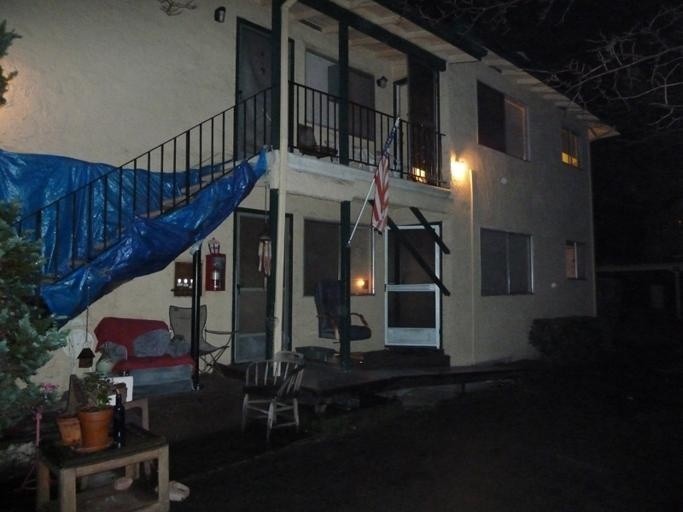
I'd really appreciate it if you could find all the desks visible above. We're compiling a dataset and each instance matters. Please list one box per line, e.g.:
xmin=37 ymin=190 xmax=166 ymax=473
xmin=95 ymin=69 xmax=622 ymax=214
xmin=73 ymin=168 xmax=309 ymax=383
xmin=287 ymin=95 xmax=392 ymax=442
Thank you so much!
xmin=35 ymin=423 xmax=170 ymax=512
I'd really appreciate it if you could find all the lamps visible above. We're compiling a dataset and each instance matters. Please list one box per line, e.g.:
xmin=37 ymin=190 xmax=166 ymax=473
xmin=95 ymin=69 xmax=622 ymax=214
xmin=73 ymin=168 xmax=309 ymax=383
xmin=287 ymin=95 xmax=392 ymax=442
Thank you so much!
xmin=257 ymin=233 xmax=272 ymax=278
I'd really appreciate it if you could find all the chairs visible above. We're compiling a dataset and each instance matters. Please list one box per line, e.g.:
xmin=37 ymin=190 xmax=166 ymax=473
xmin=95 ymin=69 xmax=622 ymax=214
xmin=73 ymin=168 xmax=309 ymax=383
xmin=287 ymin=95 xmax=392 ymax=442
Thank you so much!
xmin=297 ymin=123 xmax=338 ymax=159
xmin=240 ymin=351 xmax=309 ymax=442
xmin=169 ymin=305 xmax=235 ymax=379
xmin=314 ymin=279 xmax=371 ymax=367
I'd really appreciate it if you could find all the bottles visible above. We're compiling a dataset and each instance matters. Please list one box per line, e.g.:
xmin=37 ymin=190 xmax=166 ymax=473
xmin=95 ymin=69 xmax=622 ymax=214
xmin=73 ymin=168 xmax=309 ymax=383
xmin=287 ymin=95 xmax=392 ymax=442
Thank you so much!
xmin=114 ymin=394 xmax=125 ymax=449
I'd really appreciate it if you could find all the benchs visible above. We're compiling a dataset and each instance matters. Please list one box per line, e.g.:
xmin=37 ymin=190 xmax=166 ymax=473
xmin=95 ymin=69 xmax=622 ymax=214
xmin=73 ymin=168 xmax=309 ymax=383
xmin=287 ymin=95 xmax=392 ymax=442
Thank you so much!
xmin=94 ymin=317 xmax=193 ymax=397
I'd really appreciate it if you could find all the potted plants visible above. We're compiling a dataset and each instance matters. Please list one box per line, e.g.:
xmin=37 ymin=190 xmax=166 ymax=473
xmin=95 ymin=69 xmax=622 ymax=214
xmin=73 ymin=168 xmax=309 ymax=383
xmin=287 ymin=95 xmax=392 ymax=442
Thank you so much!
xmin=56 ymin=400 xmax=83 ymax=448
xmin=77 ymin=370 xmax=115 ymax=450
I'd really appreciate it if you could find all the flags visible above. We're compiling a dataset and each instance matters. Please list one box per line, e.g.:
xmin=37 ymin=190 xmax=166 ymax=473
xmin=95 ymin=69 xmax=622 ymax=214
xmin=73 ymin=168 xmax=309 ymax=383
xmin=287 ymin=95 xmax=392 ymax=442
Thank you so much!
xmin=371 ymin=118 xmax=400 ymax=235
